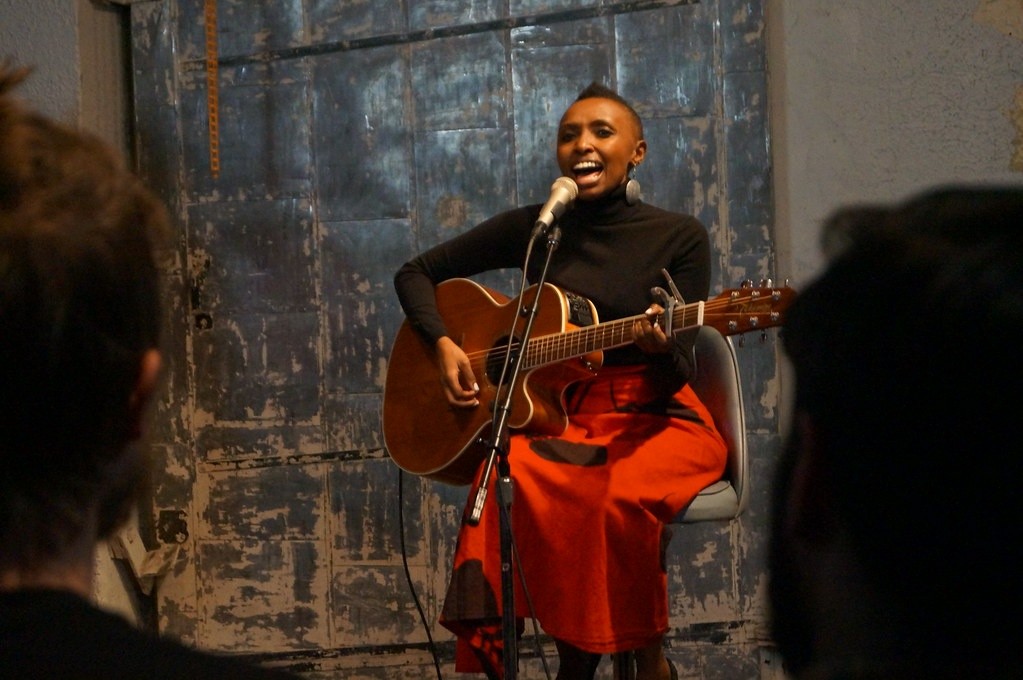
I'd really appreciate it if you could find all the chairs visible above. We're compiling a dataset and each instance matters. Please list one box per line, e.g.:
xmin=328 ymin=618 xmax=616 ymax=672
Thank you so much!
xmin=613 ymin=326 xmax=750 ymax=680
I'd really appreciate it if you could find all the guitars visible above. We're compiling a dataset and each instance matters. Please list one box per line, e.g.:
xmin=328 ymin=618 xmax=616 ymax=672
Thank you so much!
xmin=380 ymin=276 xmax=802 ymax=489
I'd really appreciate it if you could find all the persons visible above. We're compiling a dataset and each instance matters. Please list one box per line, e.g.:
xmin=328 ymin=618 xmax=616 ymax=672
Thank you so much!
xmin=394 ymin=82 xmax=729 ymax=680
xmin=765 ymin=184 xmax=1022 ymax=680
xmin=0 ymin=63 xmax=315 ymax=680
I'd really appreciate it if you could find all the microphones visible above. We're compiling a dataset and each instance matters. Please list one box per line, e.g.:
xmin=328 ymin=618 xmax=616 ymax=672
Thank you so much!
xmin=530 ymin=176 xmax=578 ymax=243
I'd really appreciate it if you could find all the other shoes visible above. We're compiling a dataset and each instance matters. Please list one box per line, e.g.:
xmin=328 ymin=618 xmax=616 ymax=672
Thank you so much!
xmin=556 ymin=653 xmax=601 ymax=680
xmin=666 ymin=657 xmax=678 ymax=680
